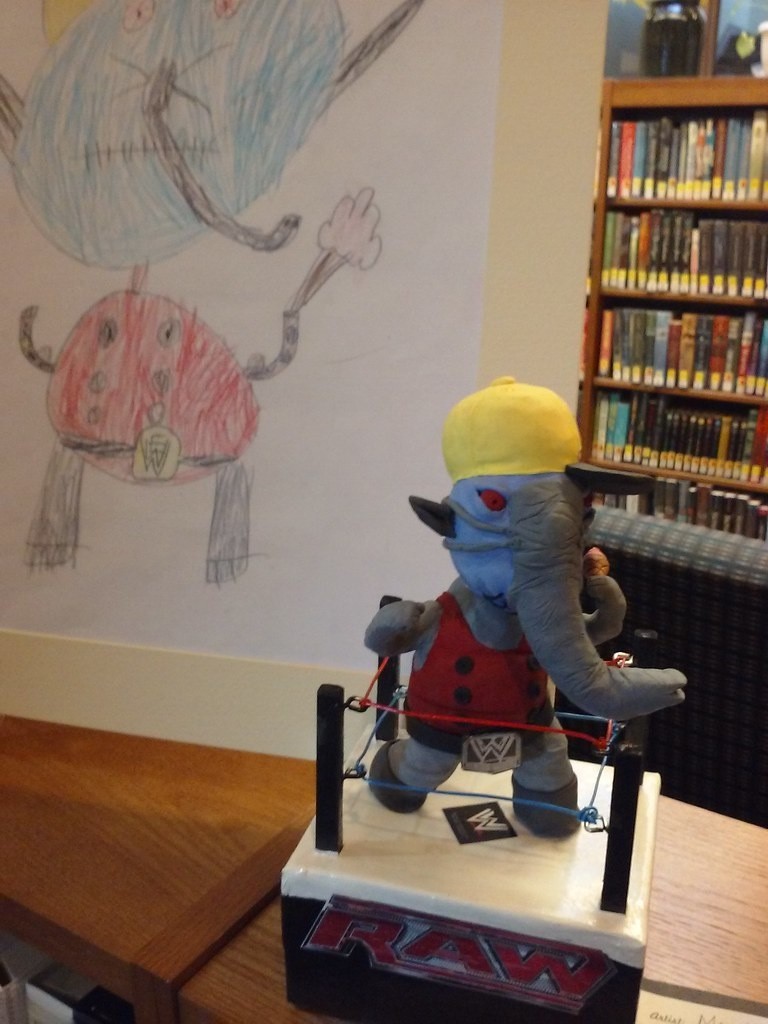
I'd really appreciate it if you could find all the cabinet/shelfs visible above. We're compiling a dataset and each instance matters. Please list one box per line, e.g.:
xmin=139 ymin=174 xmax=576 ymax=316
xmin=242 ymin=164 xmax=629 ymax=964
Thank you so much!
xmin=552 ymin=66 xmax=768 ymax=543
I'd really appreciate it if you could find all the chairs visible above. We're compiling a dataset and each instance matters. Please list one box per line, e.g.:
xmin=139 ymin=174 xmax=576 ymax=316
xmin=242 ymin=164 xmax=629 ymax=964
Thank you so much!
xmin=555 ymin=505 xmax=768 ymax=823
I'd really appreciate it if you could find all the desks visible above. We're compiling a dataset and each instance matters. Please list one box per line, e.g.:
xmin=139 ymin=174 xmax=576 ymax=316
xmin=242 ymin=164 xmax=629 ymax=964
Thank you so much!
xmin=0 ymin=708 xmax=768 ymax=1024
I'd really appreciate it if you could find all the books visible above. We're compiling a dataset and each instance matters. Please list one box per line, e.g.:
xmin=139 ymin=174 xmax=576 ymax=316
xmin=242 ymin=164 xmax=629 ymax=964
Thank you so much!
xmin=605 ymin=110 xmax=768 ymax=200
xmin=589 ymin=389 xmax=768 ymax=484
xmin=598 ymin=309 xmax=768 ymax=399
xmin=592 ymin=475 xmax=768 ymax=540
xmin=600 ymin=206 xmax=768 ymax=298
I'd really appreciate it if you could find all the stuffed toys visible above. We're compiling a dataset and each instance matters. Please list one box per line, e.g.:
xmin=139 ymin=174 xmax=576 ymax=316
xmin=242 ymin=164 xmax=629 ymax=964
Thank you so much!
xmin=364 ymin=372 xmax=689 ymax=835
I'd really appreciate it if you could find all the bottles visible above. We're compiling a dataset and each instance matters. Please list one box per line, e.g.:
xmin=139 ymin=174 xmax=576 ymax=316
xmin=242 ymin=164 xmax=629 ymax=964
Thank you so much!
xmin=638 ymin=0 xmax=707 ymax=78
